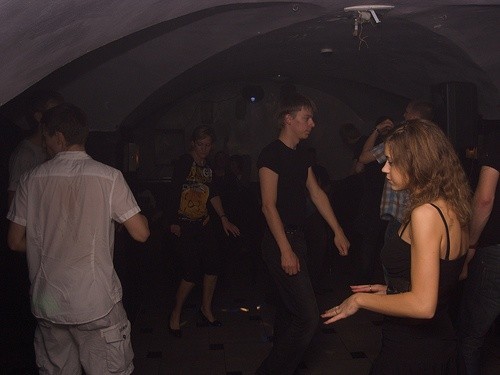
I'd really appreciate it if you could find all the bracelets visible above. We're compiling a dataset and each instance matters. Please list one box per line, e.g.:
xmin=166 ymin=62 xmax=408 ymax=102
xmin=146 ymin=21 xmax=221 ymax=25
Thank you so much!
xmin=375 ymin=128 xmax=381 ymax=133
xmin=221 ymin=215 xmax=224 ymax=217
xmin=470 ymin=245 xmax=477 ymax=249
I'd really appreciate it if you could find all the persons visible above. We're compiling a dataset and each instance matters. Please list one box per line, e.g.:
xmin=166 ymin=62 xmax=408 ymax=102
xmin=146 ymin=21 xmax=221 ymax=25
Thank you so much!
xmin=170 ymin=126 xmax=240 ymax=337
xmin=321 ymin=119 xmax=472 ymax=375
xmin=440 ymin=131 xmax=500 ymax=375
xmin=255 ymin=95 xmax=373 ymax=375
xmin=2 ymin=105 xmax=150 ymax=375
xmin=359 ymin=100 xmax=430 ymax=286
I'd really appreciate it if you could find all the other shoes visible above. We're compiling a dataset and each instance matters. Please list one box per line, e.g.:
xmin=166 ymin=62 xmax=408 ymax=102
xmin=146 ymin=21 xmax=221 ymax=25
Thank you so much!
xmin=198 ymin=307 xmax=221 ymax=328
xmin=168 ymin=319 xmax=182 ymax=338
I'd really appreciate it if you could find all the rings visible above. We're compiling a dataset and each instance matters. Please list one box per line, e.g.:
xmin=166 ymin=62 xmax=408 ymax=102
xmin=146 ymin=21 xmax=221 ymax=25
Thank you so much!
xmin=370 ymin=285 xmax=371 ymax=292
xmin=335 ymin=310 xmax=337 ymax=315
